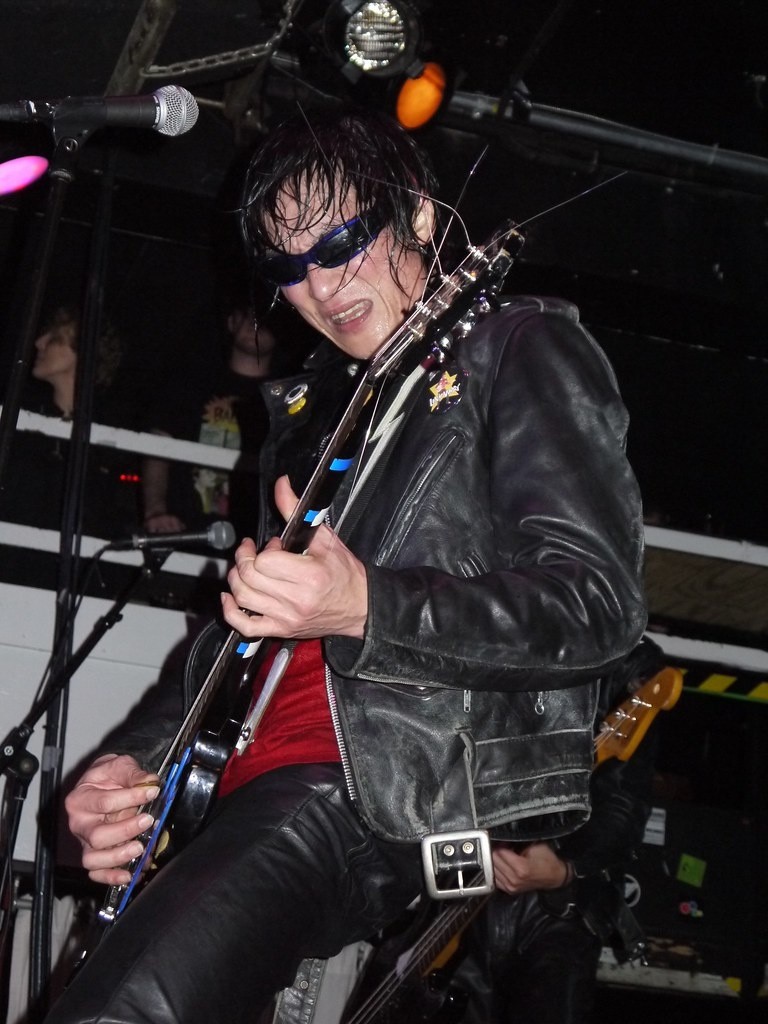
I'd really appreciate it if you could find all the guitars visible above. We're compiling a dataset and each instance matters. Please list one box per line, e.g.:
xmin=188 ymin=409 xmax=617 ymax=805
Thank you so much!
xmin=84 ymin=217 xmax=527 ymax=923
xmin=339 ymin=664 xmax=688 ymax=1023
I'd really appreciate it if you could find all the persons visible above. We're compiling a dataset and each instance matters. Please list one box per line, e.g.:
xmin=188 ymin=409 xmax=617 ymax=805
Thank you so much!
xmin=9 ymin=306 xmax=137 ymax=546
xmin=142 ymin=289 xmax=288 ymax=534
xmin=65 ymin=117 xmax=677 ymax=1024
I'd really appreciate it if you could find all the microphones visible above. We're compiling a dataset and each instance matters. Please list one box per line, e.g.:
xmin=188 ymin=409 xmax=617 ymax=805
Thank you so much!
xmin=0 ymin=84 xmax=199 ymax=136
xmin=111 ymin=520 xmax=236 ymax=551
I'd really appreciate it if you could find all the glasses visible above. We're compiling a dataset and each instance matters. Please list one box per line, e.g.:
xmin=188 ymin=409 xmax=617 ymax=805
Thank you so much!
xmin=262 ymin=191 xmax=415 ymax=287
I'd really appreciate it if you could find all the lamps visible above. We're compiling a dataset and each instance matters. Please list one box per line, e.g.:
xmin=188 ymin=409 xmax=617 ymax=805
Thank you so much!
xmin=310 ymin=0 xmax=456 ymax=139
